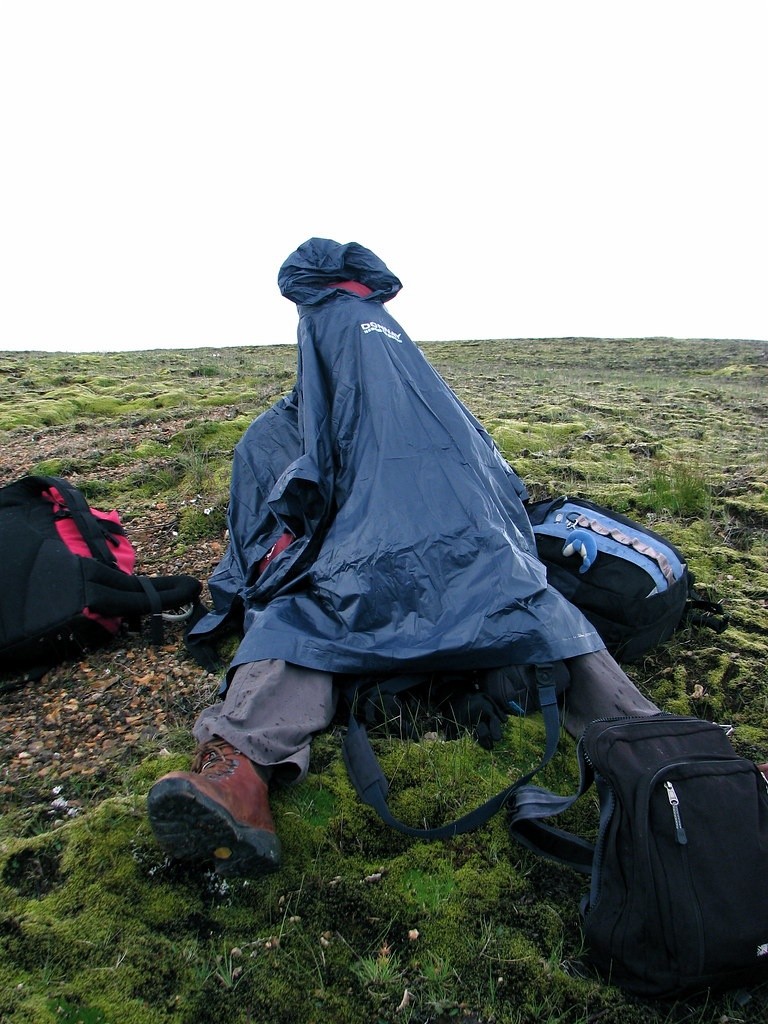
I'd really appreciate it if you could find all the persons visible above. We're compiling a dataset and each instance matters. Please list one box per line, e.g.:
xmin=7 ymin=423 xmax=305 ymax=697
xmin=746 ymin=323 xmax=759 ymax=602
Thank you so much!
xmin=147 ymin=280 xmax=768 ymax=876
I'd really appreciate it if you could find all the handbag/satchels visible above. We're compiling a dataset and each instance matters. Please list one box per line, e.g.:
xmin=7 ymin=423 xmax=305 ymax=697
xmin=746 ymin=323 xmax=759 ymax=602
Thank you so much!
xmin=342 ymin=659 xmax=570 ymax=841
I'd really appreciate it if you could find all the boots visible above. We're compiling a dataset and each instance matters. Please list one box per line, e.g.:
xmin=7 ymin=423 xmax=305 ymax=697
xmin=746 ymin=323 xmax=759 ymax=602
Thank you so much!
xmin=147 ymin=740 xmax=281 ymax=874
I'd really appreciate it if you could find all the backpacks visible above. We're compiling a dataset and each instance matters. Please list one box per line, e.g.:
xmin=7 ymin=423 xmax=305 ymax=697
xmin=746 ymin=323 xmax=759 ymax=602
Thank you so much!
xmin=529 ymin=494 xmax=692 ymax=667
xmin=505 ymin=712 xmax=768 ymax=1005
xmin=0 ymin=475 xmax=201 ymax=664
